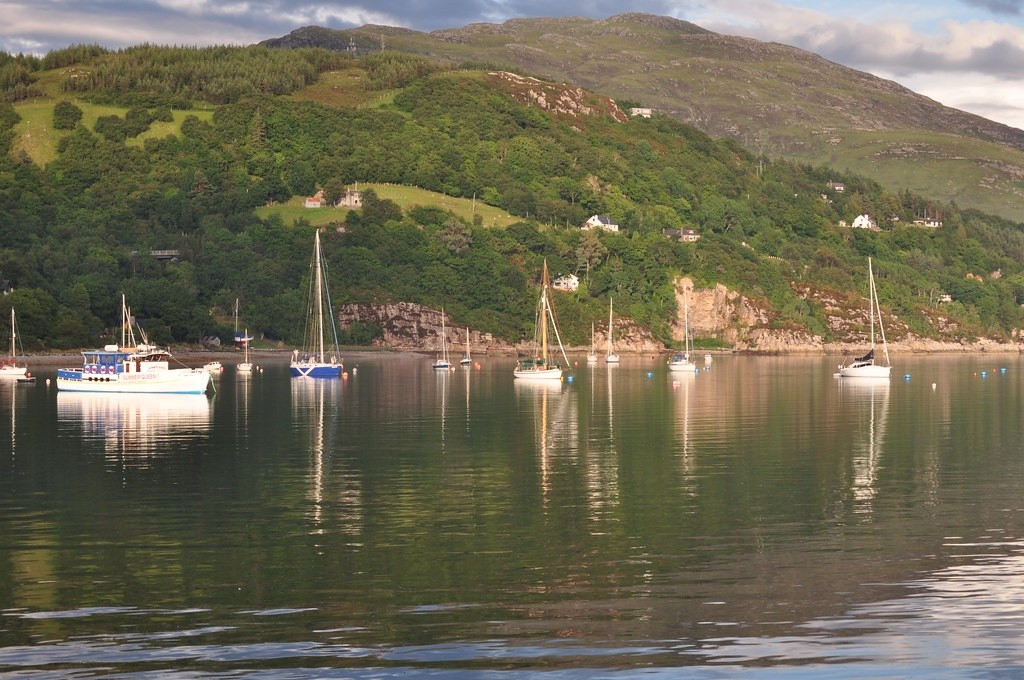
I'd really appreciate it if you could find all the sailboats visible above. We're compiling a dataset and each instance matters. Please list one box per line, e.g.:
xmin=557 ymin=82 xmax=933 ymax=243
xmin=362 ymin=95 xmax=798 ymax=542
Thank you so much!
xmin=516 ymin=255 xmax=562 ymax=370
xmin=585 ymin=321 xmax=597 ymax=361
xmin=0 ymin=305 xmax=37 ymax=383
xmin=56 ymin=295 xmax=210 ymax=393
xmin=236 ymin=328 xmax=253 ymax=371
xmin=432 ymin=308 xmax=452 ymax=371
xmin=289 ymin=228 xmax=344 ymax=378
xmin=459 ymin=326 xmax=472 ymax=365
xmin=667 ymin=280 xmax=696 ymax=371
xmin=604 ymin=296 xmax=619 ymax=362
xmin=511 ymin=287 xmax=563 ymax=378
xmin=135 ymin=321 xmax=172 ymax=360
xmin=231 ymin=299 xmax=254 ymax=342
xmin=838 ymin=255 xmax=891 ymax=378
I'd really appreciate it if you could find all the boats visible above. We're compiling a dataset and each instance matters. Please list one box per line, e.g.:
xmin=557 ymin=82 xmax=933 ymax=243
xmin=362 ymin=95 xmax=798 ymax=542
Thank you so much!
xmin=202 ymin=360 xmax=222 ymax=371
